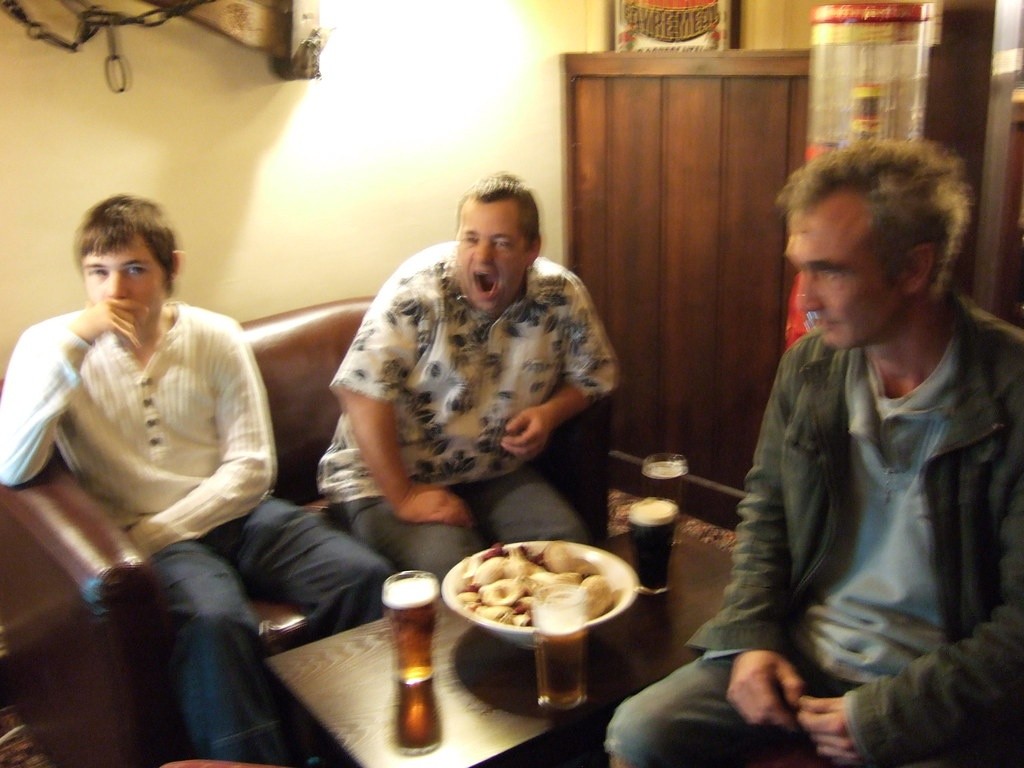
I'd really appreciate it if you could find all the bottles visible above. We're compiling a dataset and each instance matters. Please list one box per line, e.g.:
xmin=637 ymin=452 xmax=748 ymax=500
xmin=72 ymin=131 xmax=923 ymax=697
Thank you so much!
xmin=847 ymin=44 xmax=883 ymax=148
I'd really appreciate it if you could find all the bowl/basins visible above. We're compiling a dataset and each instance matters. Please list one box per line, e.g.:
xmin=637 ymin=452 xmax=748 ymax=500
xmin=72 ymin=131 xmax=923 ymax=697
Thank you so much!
xmin=441 ymin=540 xmax=640 ymax=649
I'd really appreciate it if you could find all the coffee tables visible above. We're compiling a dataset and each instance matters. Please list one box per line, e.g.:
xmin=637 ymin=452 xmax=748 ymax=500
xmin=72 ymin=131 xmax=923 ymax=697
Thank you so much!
xmin=265 ymin=493 xmax=778 ymax=767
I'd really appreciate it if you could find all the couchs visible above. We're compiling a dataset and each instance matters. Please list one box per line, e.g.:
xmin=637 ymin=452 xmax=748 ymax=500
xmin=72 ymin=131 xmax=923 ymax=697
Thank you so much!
xmin=0 ymin=295 xmax=608 ymax=767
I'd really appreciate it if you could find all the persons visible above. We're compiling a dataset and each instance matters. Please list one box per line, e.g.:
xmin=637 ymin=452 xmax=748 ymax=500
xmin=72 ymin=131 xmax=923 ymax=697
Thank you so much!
xmin=604 ymin=134 xmax=1024 ymax=768
xmin=0 ymin=196 xmax=392 ymax=768
xmin=316 ymin=171 xmax=618 ymax=586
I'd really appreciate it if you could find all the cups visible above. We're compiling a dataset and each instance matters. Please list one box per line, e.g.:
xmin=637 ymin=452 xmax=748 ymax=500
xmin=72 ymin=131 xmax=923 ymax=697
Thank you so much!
xmin=529 ymin=584 xmax=589 ymax=711
xmin=628 ymin=496 xmax=679 ymax=595
xmin=380 ymin=570 xmax=440 ymax=685
xmin=389 ymin=682 xmax=442 ymax=757
xmin=640 ymin=452 xmax=689 ymax=504
